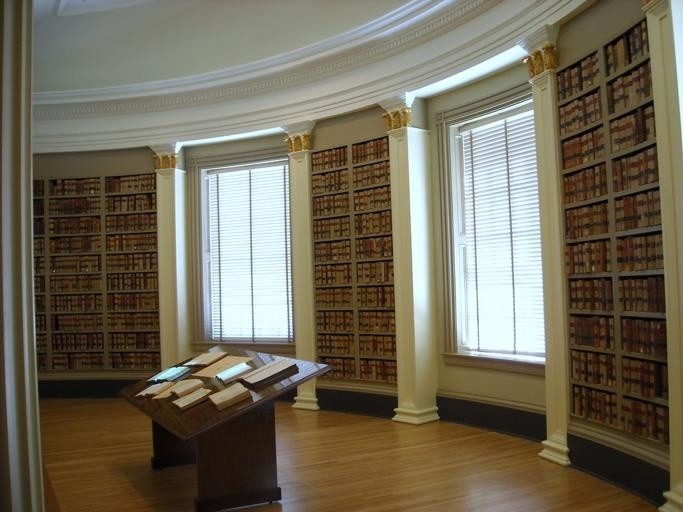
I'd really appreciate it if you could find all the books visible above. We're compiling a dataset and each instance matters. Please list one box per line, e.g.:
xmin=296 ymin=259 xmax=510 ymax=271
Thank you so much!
xmin=33 ymin=171 xmax=159 ymax=373
xmin=554 ymin=19 xmax=670 ymax=449
xmin=135 ymin=353 xmax=298 ymax=413
xmin=312 ymin=137 xmax=396 ymax=384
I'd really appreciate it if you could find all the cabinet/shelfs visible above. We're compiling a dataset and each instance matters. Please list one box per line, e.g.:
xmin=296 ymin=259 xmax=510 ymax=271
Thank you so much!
xmin=308 ymin=134 xmax=397 ymax=387
xmin=31 ymin=169 xmax=161 ymax=371
xmin=553 ymin=16 xmax=668 ymax=458
